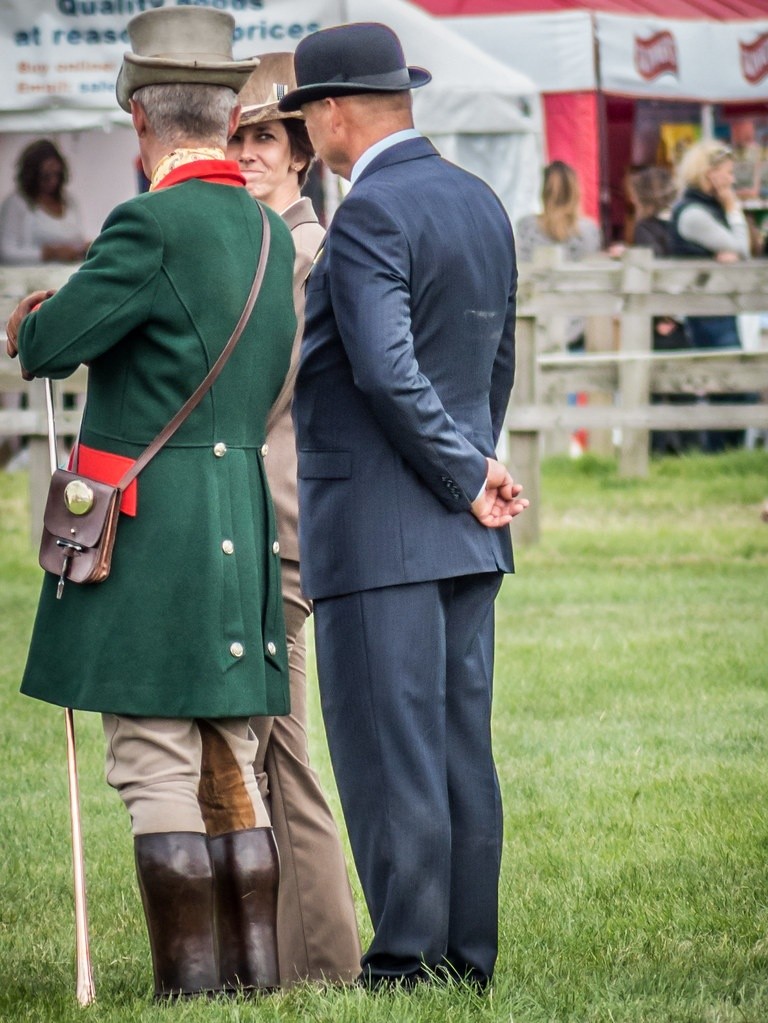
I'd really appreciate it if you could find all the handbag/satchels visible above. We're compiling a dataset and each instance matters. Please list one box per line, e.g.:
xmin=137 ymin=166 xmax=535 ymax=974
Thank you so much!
xmin=38 ymin=468 xmax=123 ymax=600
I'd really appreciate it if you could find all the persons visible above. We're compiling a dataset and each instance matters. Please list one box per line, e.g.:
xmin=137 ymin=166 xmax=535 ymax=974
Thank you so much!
xmin=354 ymin=1007 xmax=358 ymax=1010
xmin=0 ymin=7 xmax=768 ymax=1010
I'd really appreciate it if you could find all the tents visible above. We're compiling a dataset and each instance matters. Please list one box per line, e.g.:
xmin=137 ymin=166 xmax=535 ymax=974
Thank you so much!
xmin=323 ymin=0 xmax=768 ymax=254
xmin=0 ymin=0 xmax=546 ymax=216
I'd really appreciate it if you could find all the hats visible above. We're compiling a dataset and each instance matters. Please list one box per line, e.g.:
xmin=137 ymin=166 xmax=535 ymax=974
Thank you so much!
xmin=237 ymin=51 xmax=306 ymax=126
xmin=277 ymin=22 xmax=432 ymax=113
xmin=114 ymin=6 xmax=262 ymax=115
